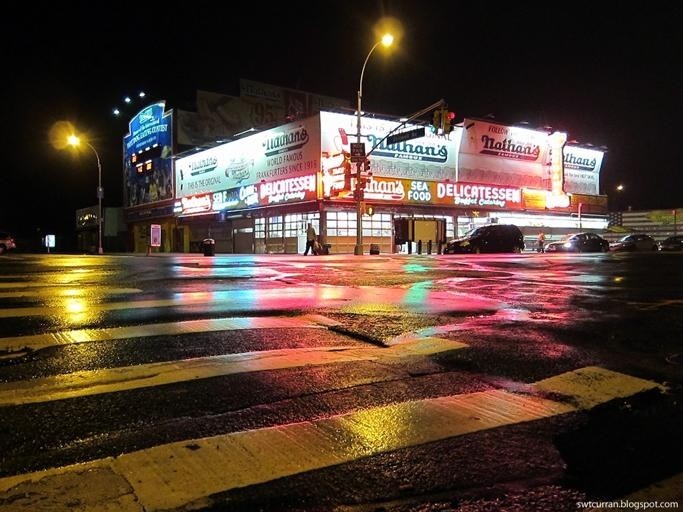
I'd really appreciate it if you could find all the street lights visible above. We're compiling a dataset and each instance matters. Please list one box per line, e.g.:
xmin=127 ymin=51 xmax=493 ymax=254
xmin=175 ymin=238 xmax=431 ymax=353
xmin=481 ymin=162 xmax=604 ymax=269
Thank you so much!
xmin=354 ymin=33 xmax=394 ymax=256
xmin=68 ymin=136 xmax=103 ymax=255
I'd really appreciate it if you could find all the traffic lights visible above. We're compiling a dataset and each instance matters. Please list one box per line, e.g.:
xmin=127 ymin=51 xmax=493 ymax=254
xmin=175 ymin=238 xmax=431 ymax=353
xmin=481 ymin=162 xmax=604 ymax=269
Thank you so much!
xmin=441 ymin=109 xmax=455 ymax=135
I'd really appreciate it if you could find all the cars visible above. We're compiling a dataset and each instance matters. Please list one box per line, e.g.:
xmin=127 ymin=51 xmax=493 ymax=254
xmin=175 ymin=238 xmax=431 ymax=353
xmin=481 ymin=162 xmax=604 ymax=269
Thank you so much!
xmin=544 ymin=232 xmax=683 ymax=253
xmin=0 ymin=231 xmax=16 ymax=254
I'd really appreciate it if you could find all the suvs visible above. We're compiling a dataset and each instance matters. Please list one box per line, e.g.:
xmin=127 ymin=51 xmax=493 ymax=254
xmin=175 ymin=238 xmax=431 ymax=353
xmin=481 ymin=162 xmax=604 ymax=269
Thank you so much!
xmin=444 ymin=224 xmax=526 ymax=254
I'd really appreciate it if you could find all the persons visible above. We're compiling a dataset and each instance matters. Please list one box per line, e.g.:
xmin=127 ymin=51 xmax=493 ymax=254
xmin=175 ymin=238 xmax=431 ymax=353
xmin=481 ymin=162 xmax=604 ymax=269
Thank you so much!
xmin=536 ymin=230 xmax=545 ymax=253
xmin=303 ymin=223 xmax=318 ymax=256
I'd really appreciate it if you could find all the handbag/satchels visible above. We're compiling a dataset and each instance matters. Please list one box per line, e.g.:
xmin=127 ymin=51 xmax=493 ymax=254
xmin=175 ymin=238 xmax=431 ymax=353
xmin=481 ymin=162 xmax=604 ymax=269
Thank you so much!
xmin=313 ymin=242 xmax=319 ymax=252
